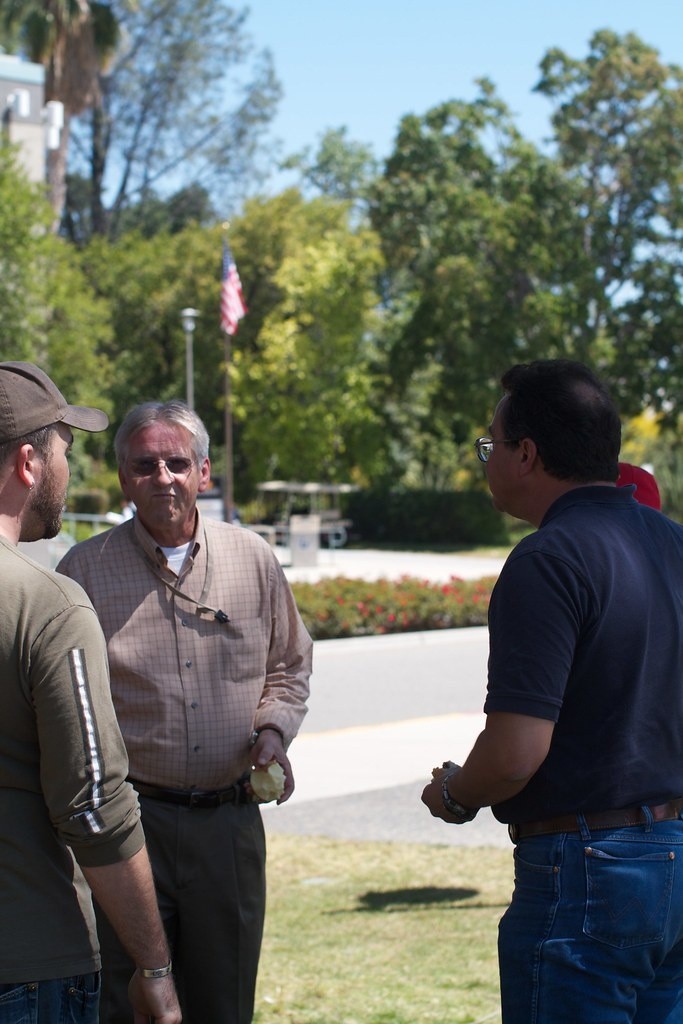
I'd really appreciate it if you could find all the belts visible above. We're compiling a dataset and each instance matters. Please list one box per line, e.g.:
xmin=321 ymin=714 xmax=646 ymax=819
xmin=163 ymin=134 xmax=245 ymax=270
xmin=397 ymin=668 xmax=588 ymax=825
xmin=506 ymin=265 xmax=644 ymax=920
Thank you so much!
xmin=508 ymin=799 xmax=683 ymax=841
xmin=127 ymin=776 xmax=249 ymax=808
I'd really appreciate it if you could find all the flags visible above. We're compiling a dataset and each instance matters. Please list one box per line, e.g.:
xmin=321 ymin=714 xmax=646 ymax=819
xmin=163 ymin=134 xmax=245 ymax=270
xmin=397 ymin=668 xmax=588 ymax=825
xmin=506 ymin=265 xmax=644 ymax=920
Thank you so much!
xmin=220 ymin=243 xmax=248 ymax=338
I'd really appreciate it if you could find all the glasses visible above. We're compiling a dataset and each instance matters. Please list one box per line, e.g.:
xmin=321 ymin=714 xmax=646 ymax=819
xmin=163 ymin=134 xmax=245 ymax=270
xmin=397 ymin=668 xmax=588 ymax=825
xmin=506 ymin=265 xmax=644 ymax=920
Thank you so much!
xmin=476 ymin=436 xmax=519 ymax=461
xmin=123 ymin=453 xmax=203 ymax=476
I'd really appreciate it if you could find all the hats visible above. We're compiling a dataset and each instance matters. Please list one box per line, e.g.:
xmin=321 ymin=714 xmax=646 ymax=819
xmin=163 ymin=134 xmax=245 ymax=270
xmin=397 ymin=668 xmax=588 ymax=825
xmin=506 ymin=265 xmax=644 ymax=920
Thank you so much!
xmin=617 ymin=463 xmax=661 ymax=511
xmin=0 ymin=360 xmax=110 ymax=442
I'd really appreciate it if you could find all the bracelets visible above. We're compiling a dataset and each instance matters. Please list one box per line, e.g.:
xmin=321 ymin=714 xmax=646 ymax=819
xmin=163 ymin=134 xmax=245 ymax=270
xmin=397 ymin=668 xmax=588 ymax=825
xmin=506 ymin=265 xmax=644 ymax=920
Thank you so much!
xmin=141 ymin=957 xmax=172 ymax=977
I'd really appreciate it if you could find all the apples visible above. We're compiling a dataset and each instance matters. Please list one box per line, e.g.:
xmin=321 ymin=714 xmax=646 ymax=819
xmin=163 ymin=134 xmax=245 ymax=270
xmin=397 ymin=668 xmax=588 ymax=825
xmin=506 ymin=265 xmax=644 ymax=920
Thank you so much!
xmin=249 ymin=761 xmax=286 ymax=800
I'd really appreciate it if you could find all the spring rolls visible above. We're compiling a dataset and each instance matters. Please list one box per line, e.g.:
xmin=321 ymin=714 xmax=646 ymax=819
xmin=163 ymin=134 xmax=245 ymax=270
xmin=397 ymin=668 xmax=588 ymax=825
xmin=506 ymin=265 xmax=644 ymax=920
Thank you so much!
xmin=432 ymin=761 xmax=460 ymax=781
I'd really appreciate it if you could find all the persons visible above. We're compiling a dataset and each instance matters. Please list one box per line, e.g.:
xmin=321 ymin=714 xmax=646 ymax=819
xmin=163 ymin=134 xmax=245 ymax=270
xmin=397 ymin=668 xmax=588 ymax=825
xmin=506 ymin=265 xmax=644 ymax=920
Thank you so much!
xmin=56 ymin=402 xmax=312 ymax=1024
xmin=420 ymin=356 xmax=683 ymax=1024
xmin=0 ymin=359 xmax=183 ymax=1024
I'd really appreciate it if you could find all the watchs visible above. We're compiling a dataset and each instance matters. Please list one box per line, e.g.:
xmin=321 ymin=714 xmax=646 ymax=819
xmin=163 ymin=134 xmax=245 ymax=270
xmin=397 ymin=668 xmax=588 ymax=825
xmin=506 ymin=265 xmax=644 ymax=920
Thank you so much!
xmin=439 ymin=774 xmax=480 ymax=822
xmin=248 ymin=726 xmax=285 ymax=746
